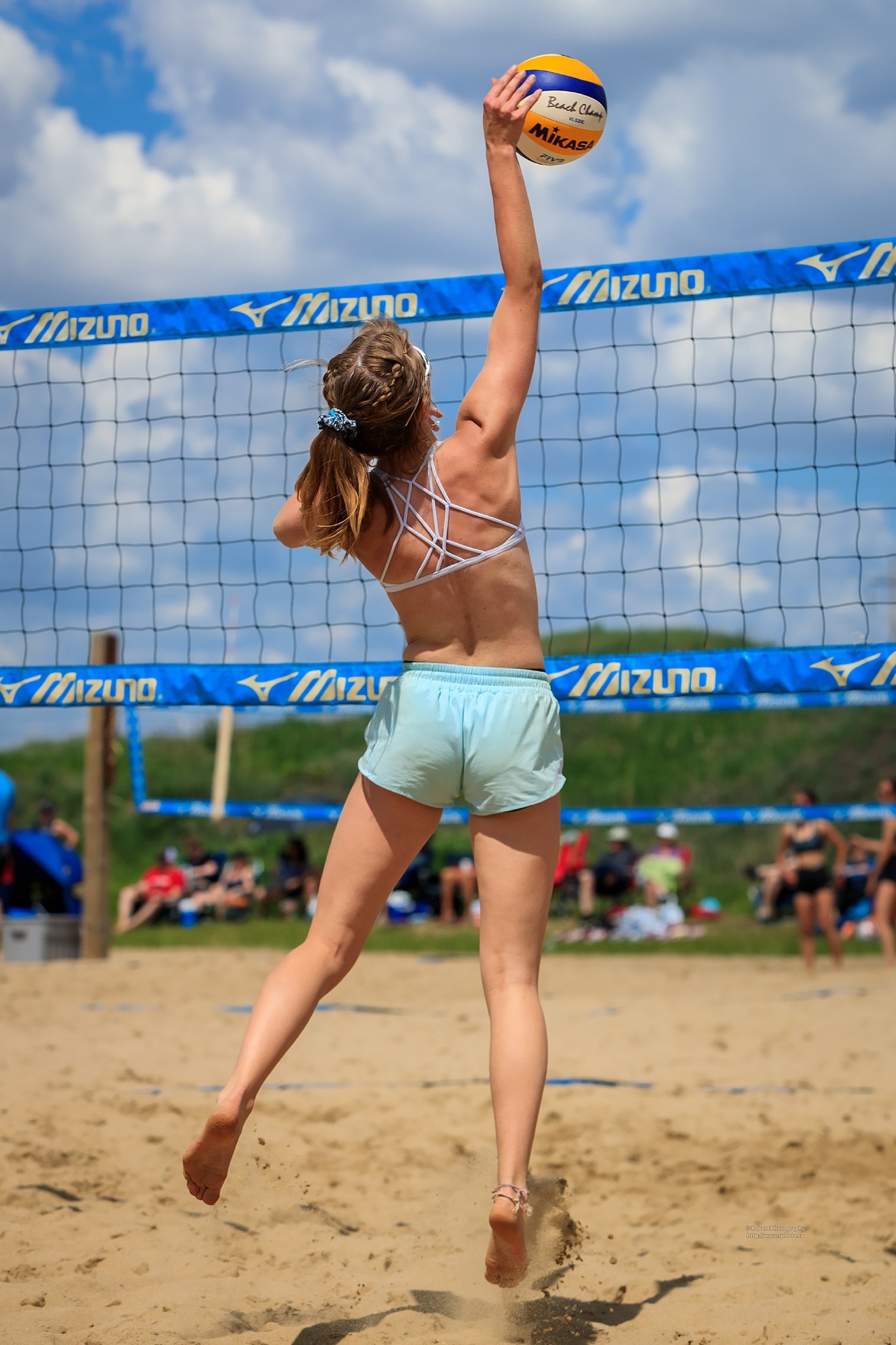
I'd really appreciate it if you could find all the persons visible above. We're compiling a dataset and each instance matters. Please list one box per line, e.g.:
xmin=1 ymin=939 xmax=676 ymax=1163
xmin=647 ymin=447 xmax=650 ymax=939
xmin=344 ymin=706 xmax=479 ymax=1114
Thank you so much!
xmin=182 ymin=64 xmax=568 ymax=1289
xmin=2 ymin=808 xmax=697 ymax=944
xmin=744 ymin=777 xmax=896 ymax=971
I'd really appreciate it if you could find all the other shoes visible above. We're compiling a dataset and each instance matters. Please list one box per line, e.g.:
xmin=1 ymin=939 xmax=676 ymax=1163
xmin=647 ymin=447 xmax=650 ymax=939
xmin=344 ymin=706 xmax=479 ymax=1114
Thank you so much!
xmin=578 ymin=909 xmax=615 ymax=929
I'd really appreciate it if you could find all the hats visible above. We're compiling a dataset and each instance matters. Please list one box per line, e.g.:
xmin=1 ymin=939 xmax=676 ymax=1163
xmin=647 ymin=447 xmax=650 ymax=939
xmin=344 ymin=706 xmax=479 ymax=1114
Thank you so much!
xmin=655 ymin=821 xmax=681 ymax=840
xmin=606 ymin=826 xmax=630 ymax=842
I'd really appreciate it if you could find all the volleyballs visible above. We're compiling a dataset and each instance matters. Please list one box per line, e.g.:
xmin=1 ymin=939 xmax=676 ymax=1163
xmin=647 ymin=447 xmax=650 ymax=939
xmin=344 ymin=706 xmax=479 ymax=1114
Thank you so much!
xmin=514 ymin=53 xmax=607 ymax=166
